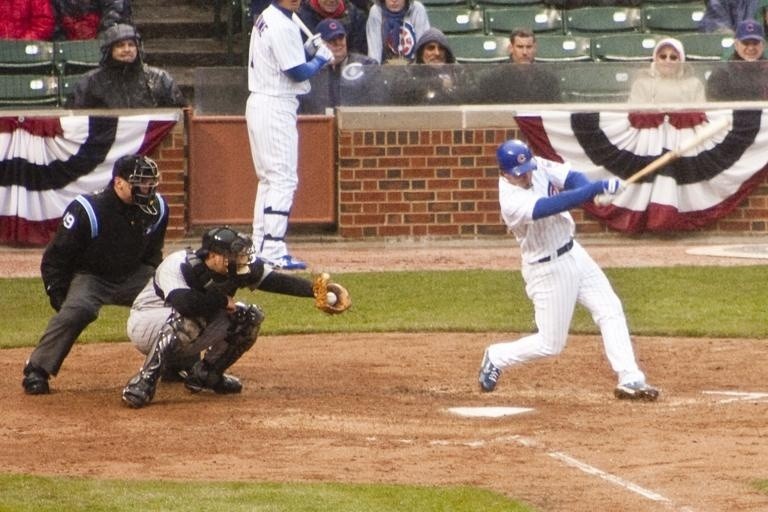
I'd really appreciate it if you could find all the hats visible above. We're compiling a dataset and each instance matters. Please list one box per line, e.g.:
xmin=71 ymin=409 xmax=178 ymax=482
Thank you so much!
xmin=316 ymin=19 xmax=346 ymax=41
xmin=736 ymin=20 xmax=764 ymax=42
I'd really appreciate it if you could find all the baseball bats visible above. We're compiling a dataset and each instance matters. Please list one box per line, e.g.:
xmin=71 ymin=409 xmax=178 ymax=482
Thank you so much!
xmin=292 ymin=13 xmax=335 ymax=64
xmin=591 ymin=117 xmax=730 ymax=209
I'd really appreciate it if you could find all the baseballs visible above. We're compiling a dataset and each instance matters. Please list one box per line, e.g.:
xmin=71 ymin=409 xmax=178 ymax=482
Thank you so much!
xmin=326 ymin=292 xmax=337 ymax=305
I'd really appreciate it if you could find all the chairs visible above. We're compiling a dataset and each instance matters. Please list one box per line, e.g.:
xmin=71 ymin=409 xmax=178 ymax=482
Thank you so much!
xmin=415 ymin=1 xmax=768 ymax=104
xmin=0 ymin=39 xmax=102 ymax=109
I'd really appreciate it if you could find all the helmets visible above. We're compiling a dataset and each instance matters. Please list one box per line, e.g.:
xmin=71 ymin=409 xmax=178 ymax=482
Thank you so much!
xmin=195 ymin=225 xmax=256 ymax=287
xmin=112 ymin=154 xmax=161 ymax=217
xmin=100 ymin=23 xmax=141 ymax=47
xmin=497 ymin=139 xmax=537 ymax=176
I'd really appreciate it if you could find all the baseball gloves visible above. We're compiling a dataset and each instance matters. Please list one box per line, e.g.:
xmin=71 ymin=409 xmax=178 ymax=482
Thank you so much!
xmin=311 ymin=271 xmax=353 ymax=315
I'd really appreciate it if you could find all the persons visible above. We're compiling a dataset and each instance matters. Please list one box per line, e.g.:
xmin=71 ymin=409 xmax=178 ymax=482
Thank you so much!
xmin=297 ymin=1 xmax=562 ymax=106
xmin=245 ymin=0 xmax=334 ymax=270
xmin=22 ymin=155 xmax=170 ymax=395
xmin=0 ymin=1 xmax=189 ymax=111
xmin=121 ymin=226 xmax=352 ymax=410
xmin=476 ymin=139 xmax=658 ymax=403
xmin=627 ymin=1 xmax=767 ymax=104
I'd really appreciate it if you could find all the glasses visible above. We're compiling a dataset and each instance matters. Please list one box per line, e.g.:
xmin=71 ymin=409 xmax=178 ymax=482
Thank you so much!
xmin=660 ymin=55 xmax=677 ymax=60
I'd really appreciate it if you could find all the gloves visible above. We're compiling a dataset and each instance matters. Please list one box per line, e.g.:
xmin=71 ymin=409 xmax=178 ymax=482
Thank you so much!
xmin=48 ymin=279 xmax=70 ymax=312
xmin=594 ymin=175 xmax=627 ymax=207
xmin=303 ymin=33 xmax=334 ymax=67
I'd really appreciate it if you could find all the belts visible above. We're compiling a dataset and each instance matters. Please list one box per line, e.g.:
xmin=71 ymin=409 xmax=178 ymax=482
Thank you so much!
xmin=538 ymin=239 xmax=573 ymax=262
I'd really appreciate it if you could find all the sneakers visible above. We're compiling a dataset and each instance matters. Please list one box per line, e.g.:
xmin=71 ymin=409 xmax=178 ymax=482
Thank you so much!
xmin=122 ymin=367 xmax=242 ymax=408
xmin=479 ymin=348 xmax=501 ymax=392
xmin=268 ymin=257 xmax=307 ymax=270
xmin=614 ymin=382 xmax=658 ymax=401
xmin=22 ymin=361 xmax=49 ymax=394
